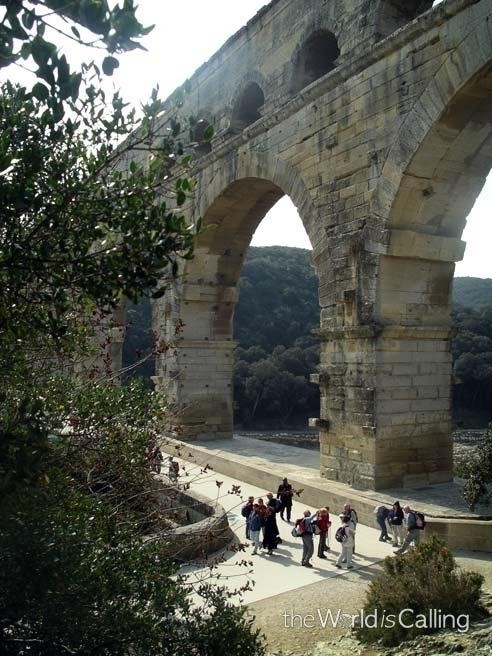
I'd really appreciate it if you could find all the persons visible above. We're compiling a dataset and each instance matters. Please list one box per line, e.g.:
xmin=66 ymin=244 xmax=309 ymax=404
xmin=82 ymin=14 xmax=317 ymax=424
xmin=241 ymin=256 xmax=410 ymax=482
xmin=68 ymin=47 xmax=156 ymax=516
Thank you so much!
xmin=388 ymin=501 xmax=404 ymax=547
xmin=392 ymin=505 xmax=420 ymax=554
xmin=277 ymin=477 xmax=293 ymax=522
xmin=241 ymin=493 xmax=282 ymax=555
xmin=302 ymin=503 xmax=358 ymax=568
xmin=374 ymin=506 xmax=392 ymax=542
xmin=152 ymin=447 xmax=164 ymax=474
xmin=169 ymin=457 xmax=179 ymax=482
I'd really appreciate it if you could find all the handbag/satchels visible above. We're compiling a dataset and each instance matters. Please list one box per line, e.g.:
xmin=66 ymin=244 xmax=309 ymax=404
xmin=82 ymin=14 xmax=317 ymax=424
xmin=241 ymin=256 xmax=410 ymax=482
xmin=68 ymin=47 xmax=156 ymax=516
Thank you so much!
xmin=275 ymin=536 xmax=282 ymax=543
xmin=292 ymin=527 xmax=298 ymax=537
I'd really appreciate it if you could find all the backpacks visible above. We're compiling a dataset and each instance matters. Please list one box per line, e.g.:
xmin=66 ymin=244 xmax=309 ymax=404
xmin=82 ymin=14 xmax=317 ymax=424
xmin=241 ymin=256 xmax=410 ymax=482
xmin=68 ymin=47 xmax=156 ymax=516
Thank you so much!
xmin=296 ymin=520 xmax=305 ymax=535
xmin=409 ymin=511 xmax=425 ymax=529
xmin=335 ymin=525 xmax=349 ymax=542
xmin=313 ymin=523 xmax=321 ymax=535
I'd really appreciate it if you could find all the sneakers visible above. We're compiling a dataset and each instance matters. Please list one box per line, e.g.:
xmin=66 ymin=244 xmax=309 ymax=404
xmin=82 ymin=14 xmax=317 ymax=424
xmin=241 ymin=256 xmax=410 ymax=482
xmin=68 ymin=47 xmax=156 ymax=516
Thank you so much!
xmin=385 ymin=536 xmax=392 ymax=540
xmin=302 ymin=545 xmax=360 ymax=568
xmin=251 ymin=550 xmax=257 ymax=555
xmin=393 ymin=549 xmax=401 ymax=555
xmin=379 ymin=537 xmax=386 ymax=542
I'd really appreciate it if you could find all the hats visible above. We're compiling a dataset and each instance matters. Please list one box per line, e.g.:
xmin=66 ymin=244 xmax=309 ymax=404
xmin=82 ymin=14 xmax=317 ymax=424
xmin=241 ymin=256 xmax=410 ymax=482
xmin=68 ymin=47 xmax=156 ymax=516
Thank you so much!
xmin=248 ymin=496 xmax=254 ymax=501
xmin=267 ymin=492 xmax=273 ymax=496
